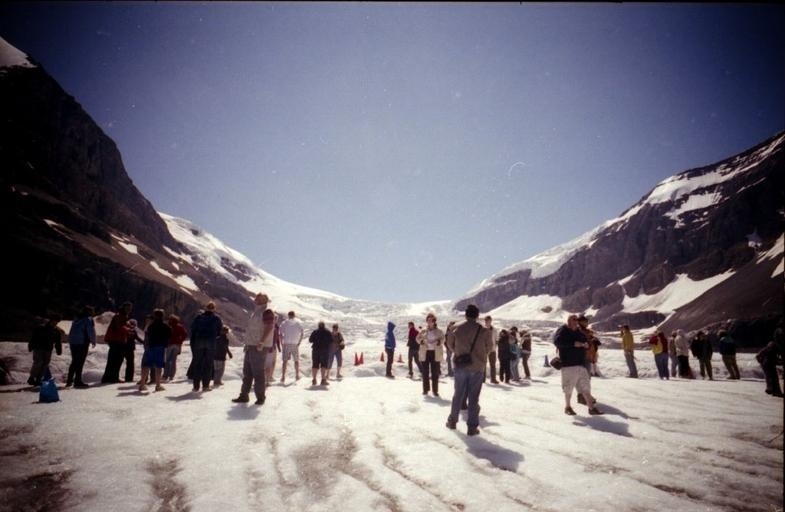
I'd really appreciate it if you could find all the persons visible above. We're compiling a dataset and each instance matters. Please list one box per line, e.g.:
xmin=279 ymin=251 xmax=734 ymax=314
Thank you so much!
xmin=553 ymin=315 xmax=600 ymax=414
xmin=717 ymin=330 xmax=740 ymax=380
xmin=674 ymin=329 xmax=696 ymax=379
xmin=385 ymin=321 xmax=396 ymax=378
xmin=309 ymin=322 xmax=335 ymax=385
xmin=406 ymin=322 xmax=422 ymax=378
xmin=232 ymin=293 xmax=275 ymax=404
xmin=497 ymin=326 xmax=521 ymax=383
xmin=416 ymin=314 xmax=445 ymax=397
xmin=119 ymin=318 xmax=144 ymax=381
xmin=446 ymin=305 xmax=494 ymax=436
xmin=326 ymin=324 xmax=345 ymax=377
xmin=65 ymin=307 xmax=96 ymax=390
xmin=444 ymin=322 xmax=457 ymax=377
xmin=620 ymin=324 xmax=638 ymax=378
xmin=670 ymin=330 xmax=680 ymax=377
xmin=137 ymin=308 xmax=174 ymax=392
xmin=757 ymin=329 xmax=784 ymax=399
xmin=649 ymin=331 xmax=670 ymax=380
xmin=577 ymin=316 xmax=597 ymax=405
xmin=190 ymin=301 xmax=232 ymax=392
xmin=162 ymin=314 xmax=187 ymax=380
xmin=280 ymin=311 xmax=304 ymax=381
xmin=690 ymin=331 xmax=714 ymax=380
xmin=519 ymin=330 xmax=531 ymax=380
xmin=590 ymin=331 xmax=602 ymax=377
xmin=482 ymin=316 xmax=499 ymax=384
xmin=27 ymin=312 xmax=63 ymax=388
xmin=101 ymin=303 xmax=132 ymax=384
xmin=265 ymin=313 xmax=281 ymax=382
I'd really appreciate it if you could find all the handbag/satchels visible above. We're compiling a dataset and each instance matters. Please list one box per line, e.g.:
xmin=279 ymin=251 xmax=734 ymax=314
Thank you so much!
xmin=455 ymin=354 xmax=473 ymax=368
xmin=550 ymin=357 xmax=561 ymax=370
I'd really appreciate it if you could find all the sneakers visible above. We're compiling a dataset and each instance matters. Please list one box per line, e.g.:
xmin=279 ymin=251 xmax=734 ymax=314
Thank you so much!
xmin=565 ymin=408 xmax=576 ymax=415
xmin=589 ymin=407 xmax=602 ymax=414
xmin=233 ymin=398 xmax=249 ymax=402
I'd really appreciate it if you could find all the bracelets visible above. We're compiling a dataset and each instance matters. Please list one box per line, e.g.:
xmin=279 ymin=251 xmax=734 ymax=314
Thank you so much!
xmin=259 ymin=342 xmax=264 ymax=344
